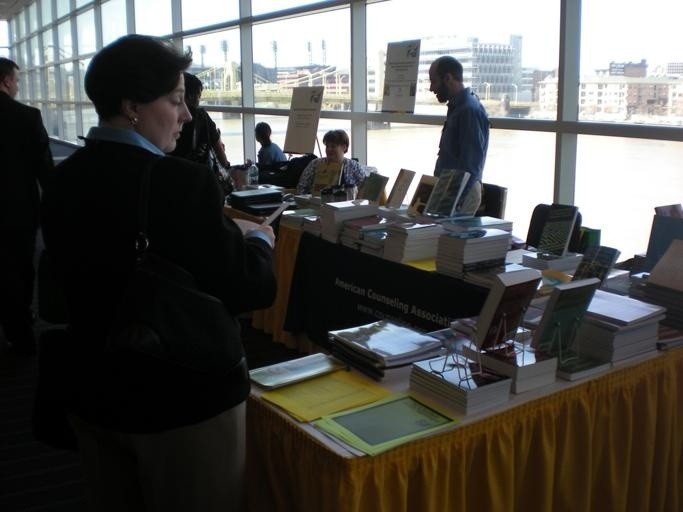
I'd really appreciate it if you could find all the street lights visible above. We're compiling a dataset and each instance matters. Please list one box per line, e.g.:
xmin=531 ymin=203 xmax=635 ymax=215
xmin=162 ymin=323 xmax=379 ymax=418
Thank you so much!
xmin=512 ymin=84 xmax=517 ymax=103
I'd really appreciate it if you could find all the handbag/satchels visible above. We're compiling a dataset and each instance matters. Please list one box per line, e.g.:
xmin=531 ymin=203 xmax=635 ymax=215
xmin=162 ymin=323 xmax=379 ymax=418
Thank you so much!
xmin=187 ymin=112 xmax=213 ymax=167
xmin=106 ymin=266 xmax=252 ymax=434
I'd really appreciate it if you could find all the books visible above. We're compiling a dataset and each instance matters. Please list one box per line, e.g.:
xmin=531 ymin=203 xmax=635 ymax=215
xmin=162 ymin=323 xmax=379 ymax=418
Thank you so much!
xmin=229 ymin=167 xmax=683 ymax=458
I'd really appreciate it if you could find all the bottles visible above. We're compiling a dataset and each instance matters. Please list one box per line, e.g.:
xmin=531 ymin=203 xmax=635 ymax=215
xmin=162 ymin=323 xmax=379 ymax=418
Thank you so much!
xmin=250 ymin=162 xmax=259 ymax=185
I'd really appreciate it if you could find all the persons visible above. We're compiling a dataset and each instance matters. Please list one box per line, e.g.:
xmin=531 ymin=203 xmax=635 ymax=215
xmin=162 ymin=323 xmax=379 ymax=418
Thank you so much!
xmin=428 ymin=56 xmax=491 ymax=215
xmin=0 ymin=34 xmax=278 ymax=512
xmin=254 ymin=122 xmax=287 ymax=164
xmin=296 ymin=129 xmax=367 ymax=195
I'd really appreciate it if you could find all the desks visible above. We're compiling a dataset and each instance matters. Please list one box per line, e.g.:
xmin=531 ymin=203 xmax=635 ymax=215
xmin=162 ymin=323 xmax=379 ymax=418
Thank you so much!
xmin=221 ymin=183 xmax=683 ymax=511
